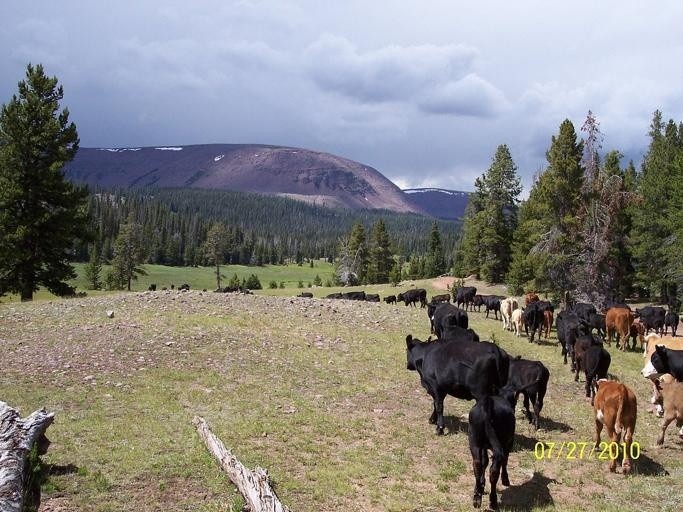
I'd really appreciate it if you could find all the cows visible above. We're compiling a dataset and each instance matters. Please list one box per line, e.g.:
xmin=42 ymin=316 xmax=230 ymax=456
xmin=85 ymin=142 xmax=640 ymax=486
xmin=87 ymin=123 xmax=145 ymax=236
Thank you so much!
xmin=382 ymin=295 xmax=396 ymax=305
xmin=426 ymin=302 xmax=468 ymax=339
xmin=431 ymin=294 xmax=450 ymax=303
xmin=468 ymin=395 xmax=516 ymax=511
xmin=405 ymin=335 xmax=510 ymax=435
xmin=455 ymin=286 xmax=554 ymax=345
xmin=397 ymin=289 xmax=426 ymax=308
xmin=325 ymin=291 xmax=380 ymax=302
xmin=508 ymin=355 xmax=550 ymax=431
xmin=441 ymin=325 xmax=479 ymax=343
xmin=554 ymin=300 xmax=682 ymax=474
xmin=296 ymin=292 xmax=313 ymax=298
